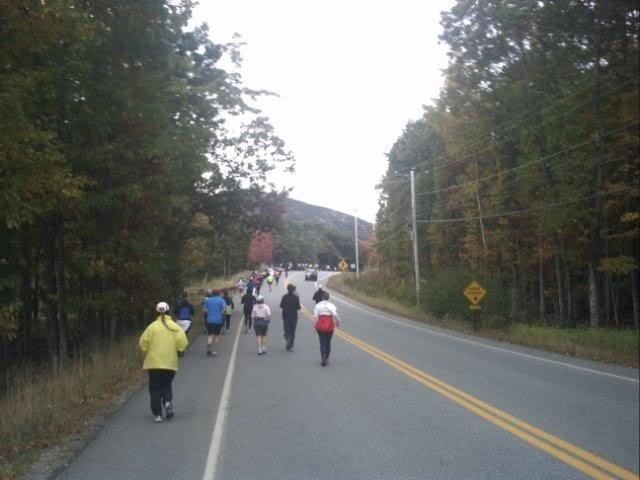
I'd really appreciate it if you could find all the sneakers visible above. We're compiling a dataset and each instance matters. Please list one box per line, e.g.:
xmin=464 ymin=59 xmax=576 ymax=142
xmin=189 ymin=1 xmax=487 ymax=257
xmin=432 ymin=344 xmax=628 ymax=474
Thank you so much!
xmin=152 ymin=415 xmax=162 ymax=423
xmin=165 ymin=401 xmax=173 ymax=420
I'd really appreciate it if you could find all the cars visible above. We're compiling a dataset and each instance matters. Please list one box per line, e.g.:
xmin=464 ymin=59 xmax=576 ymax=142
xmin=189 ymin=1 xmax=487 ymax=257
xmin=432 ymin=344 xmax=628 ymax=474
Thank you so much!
xmin=304 ymin=269 xmax=318 ymax=281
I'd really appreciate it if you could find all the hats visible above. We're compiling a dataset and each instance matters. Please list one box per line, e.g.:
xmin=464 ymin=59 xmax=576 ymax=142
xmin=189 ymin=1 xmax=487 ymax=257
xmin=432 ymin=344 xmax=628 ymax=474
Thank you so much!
xmin=155 ymin=301 xmax=170 ymax=313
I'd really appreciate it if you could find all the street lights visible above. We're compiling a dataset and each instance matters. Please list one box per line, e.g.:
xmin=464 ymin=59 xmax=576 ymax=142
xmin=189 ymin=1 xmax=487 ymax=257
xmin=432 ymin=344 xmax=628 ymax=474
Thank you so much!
xmin=352 ymin=203 xmax=362 ymax=280
xmin=314 ymin=238 xmax=321 ymax=265
xmin=394 ymin=166 xmax=421 ymax=305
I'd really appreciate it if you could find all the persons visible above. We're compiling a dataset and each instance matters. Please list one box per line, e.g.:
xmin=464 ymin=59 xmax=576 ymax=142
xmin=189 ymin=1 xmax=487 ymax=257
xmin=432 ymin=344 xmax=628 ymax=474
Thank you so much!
xmin=139 ymin=302 xmax=188 ymax=422
xmin=176 ymin=263 xmax=340 ymax=365
